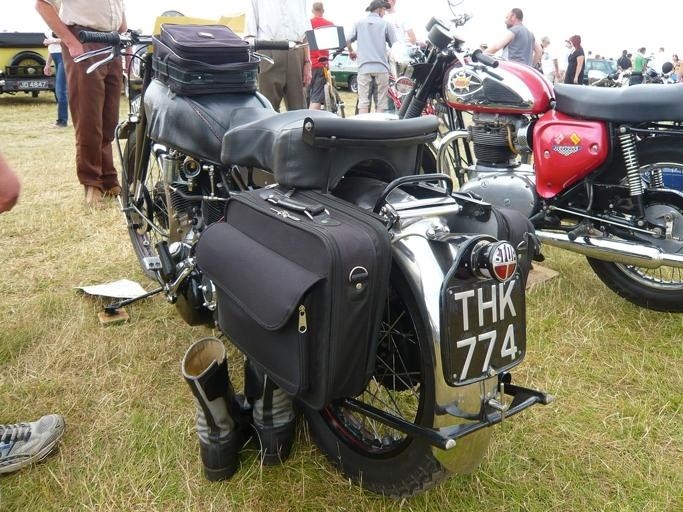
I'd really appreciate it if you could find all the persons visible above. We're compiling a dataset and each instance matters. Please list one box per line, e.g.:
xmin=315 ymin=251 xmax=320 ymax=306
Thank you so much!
xmin=34 ymin=0 xmax=134 ymax=210
xmin=309 ymin=1 xmax=334 ymax=109
xmin=468 ymin=8 xmax=683 ymax=86
xmin=0 ymin=152 xmax=66 ymax=475
xmin=244 ymin=0 xmax=312 ymax=110
xmin=345 ymin=0 xmax=425 ymax=115
xmin=43 ymin=27 xmax=69 ymax=128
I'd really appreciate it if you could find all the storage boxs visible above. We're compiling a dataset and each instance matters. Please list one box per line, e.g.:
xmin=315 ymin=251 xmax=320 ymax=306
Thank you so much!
xmin=642 ymin=167 xmax=683 ymax=192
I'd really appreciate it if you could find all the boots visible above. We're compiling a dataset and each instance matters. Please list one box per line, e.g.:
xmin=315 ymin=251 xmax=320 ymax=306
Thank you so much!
xmin=242 ymin=356 xmax=296 ymax=467
xmin=181 ymin=336 xmax=253 ymax=483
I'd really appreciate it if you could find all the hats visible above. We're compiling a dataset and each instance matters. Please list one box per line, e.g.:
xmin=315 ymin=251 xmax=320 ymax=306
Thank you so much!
xmin=365 ymin=0 xmax=390 ymax=11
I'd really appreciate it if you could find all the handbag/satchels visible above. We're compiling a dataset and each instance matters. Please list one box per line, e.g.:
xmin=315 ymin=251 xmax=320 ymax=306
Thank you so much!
xmin=194 ymin=181 xmax=392 ymax=412
xmin=151 ymin=23 xmax=261 ymax=74
xmin=152 ymin=54 xmax=262 ymax=100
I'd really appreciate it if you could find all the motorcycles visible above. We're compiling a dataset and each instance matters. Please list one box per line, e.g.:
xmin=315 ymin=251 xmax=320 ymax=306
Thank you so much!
xmin=590 ymin=68 xmax=664 ymax=89
xmin=395 ymin=0 xmax=683 ymax=313
xmin=73 ymin=22 xmax=549 ymax=501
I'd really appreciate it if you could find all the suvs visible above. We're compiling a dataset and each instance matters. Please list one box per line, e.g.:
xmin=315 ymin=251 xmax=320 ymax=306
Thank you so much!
xmin=0 ymin=33 xmax=59 ymax=103
xmin=583 ymin=56 xmax=618 ymax=86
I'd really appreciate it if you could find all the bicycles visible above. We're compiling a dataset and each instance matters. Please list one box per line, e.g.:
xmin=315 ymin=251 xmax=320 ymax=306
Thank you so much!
xmin=305 ymin=52 xmax=349 ymax=119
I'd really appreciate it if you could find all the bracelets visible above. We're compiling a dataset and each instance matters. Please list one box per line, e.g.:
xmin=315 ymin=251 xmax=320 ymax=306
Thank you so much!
xmin=304 ymin=61 xmax=311 ymax=64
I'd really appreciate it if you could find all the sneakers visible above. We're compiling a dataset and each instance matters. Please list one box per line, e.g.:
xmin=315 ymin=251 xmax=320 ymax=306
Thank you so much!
xmin=0 ymin=413 xmax=65 ymax=475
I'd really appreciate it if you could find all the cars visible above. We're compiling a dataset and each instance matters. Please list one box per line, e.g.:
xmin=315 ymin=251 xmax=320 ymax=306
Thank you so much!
xmin=120 ymin=36 xmax=150 ymax=99
xmin=329 ymin=50 xmax=395 ymax=94
